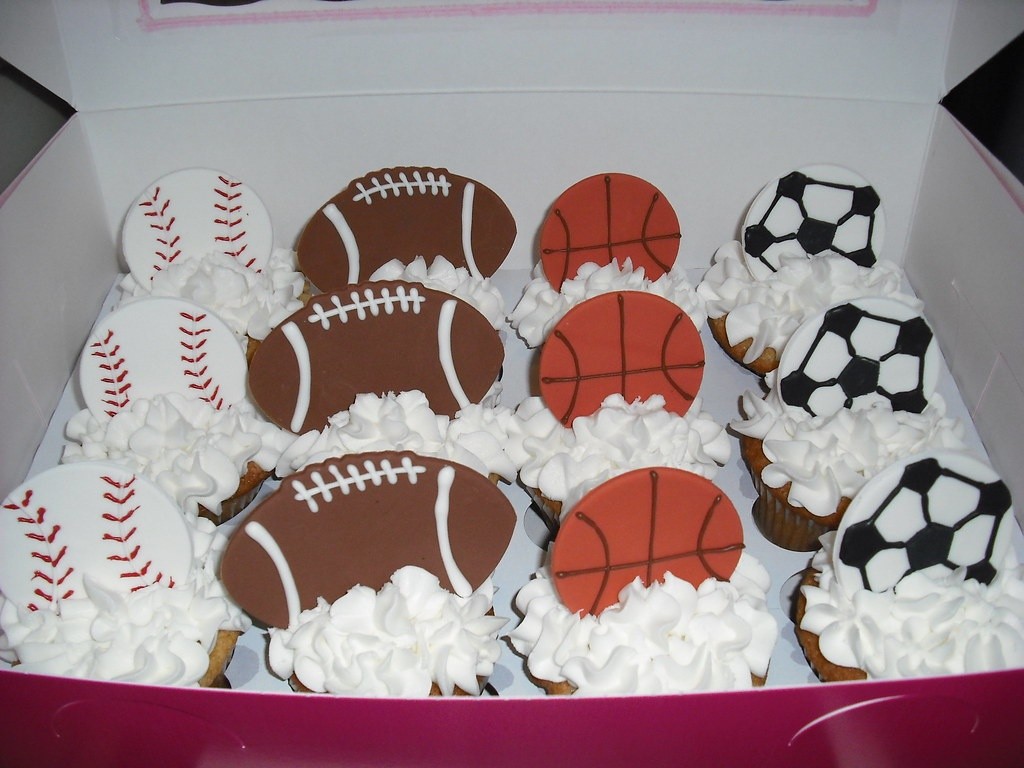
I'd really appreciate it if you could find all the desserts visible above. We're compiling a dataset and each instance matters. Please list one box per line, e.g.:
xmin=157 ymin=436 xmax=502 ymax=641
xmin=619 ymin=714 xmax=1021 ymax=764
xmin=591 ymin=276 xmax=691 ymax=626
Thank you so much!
xmin=1 ymin=165 xmax=1024 ymax=696
